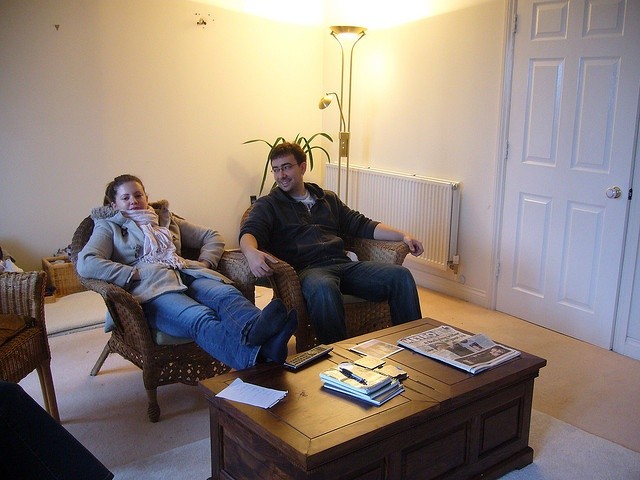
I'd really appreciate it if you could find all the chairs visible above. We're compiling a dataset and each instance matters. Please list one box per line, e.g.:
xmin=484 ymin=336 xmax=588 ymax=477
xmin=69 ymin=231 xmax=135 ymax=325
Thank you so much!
xmin=0 ymin=271 xmax=62 ymax=423
xmin=240 ymin=202 xmax=410 ymax=356
xmin=71 ymin=205 xmax=257 ymax=423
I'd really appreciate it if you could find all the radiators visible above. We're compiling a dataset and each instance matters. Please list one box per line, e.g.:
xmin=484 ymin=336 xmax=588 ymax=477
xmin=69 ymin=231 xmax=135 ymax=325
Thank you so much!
xmin=325 ymin=162 xmax=461 ymax=273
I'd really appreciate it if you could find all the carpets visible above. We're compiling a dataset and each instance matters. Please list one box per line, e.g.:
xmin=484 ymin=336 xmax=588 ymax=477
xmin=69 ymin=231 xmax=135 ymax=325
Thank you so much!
xmin=110 ymin=406 xmax=640 ymax=480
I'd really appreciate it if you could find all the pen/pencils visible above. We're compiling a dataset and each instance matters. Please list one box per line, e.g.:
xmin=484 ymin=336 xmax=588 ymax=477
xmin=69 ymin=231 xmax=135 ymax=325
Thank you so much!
xmin=340 ymin=368 xmax=368 ymax=386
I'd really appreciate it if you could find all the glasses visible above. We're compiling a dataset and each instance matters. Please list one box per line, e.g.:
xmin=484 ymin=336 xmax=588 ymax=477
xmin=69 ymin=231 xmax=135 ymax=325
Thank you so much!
xmin=270 ymin=162 xmax=301 ymax=174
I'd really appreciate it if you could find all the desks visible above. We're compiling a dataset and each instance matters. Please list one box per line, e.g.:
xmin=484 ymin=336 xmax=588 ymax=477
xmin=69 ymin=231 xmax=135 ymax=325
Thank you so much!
xmin=0 ymin=381 xmax=114 ymax=479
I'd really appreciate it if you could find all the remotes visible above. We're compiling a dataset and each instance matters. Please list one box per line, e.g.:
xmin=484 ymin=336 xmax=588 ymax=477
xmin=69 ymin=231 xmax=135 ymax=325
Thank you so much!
xmin=283 ymin=343 xmax=333 ymax=373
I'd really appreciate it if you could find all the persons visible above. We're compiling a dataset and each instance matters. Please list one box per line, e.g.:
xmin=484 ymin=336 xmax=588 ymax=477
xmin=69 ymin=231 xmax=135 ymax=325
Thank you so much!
xmin=74 ymin=173 xmax=300 ymax=370
xmin=238 ymin=140 xmax=423 ymax=344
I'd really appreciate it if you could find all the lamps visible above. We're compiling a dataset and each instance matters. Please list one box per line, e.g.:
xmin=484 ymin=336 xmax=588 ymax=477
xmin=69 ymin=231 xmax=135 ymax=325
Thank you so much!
xmin=318 ymin=25 xmax=365 ymax=208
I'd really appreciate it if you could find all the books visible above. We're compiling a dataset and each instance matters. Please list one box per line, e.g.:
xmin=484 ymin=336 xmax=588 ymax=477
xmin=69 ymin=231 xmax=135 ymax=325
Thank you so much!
xmin=319 ymin=354 xmax=407 ymax=407
